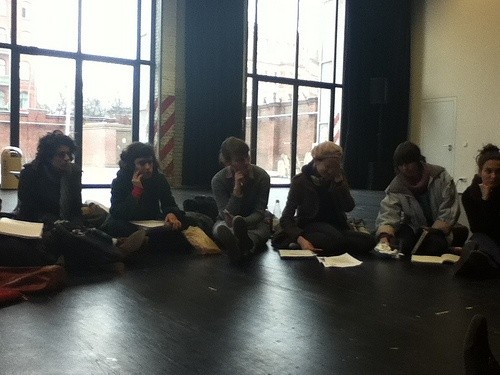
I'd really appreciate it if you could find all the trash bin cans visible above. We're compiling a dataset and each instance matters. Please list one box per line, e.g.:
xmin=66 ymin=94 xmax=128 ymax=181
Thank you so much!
xmin=1 ymin=147 xmax=23 ymax=189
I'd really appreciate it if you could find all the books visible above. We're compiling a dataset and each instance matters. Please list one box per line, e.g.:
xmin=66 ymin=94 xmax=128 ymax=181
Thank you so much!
xmin=128 ymin=219 xmax=173 ymax=231
xmin=0 ymin=216 xmax=44 ymax=240
xmin=410 ymin=254 xmax=461 ymax=265
xmin=277 ymin=249 xmax=317 ymax=261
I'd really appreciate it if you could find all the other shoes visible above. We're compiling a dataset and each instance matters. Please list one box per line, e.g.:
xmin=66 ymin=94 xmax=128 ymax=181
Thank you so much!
xmin=462 ymin=313 xmax=495 ymax=375
xmin=213 ymin=215 xmax=253 ymax=249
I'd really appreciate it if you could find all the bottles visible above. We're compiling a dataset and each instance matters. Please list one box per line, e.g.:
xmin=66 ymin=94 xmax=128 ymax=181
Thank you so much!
xmin=273 ymin=200 xmax=281 ymax=219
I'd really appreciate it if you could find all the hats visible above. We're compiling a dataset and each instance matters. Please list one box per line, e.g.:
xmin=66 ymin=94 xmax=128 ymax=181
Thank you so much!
xmin=312 ymin=142 xmax=342 ymax=159
xmin=394 ymin=141 xmax=423 ymax=164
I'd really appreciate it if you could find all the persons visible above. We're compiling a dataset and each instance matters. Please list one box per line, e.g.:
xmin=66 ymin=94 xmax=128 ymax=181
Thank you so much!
xmin=462 ymin=312 xmax=500 ymax=375
xmin=375 ymin=140 xmax=468 ymax=256
xmin=210 ymin=137 xmax=275 ymax=264
xmin=462 ymin=145 xmax=500 ymax=283
xmin=103 ymin=144 xmax=195 ymax=267
xmin=12 ymin=131 xmax=146 ymax=277
xmin=278 ymin=140 xmax=375 ymax=256
